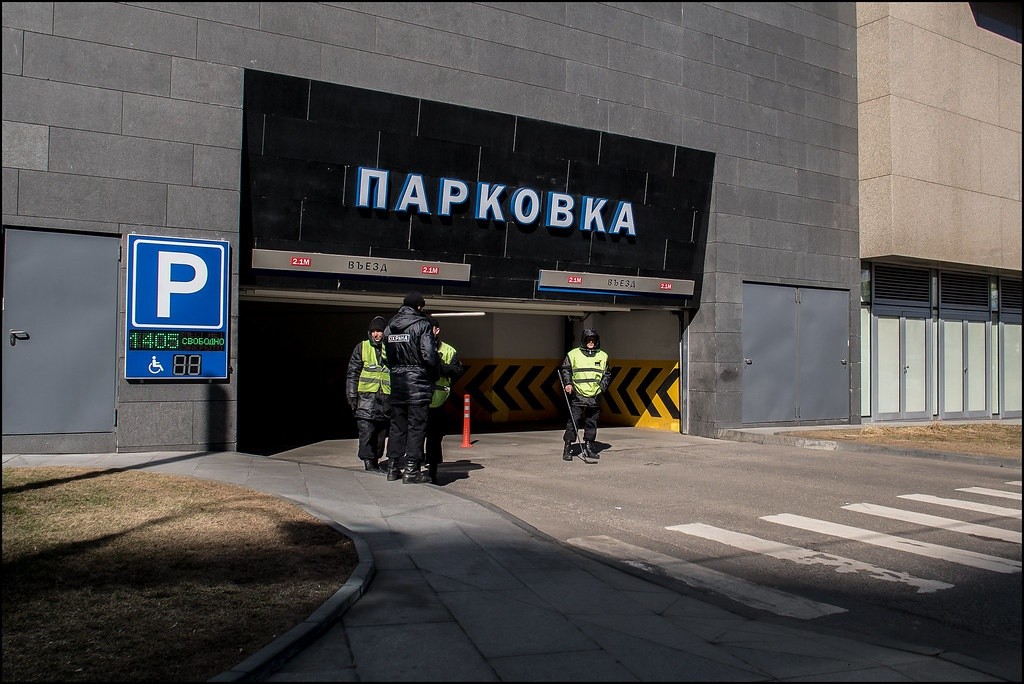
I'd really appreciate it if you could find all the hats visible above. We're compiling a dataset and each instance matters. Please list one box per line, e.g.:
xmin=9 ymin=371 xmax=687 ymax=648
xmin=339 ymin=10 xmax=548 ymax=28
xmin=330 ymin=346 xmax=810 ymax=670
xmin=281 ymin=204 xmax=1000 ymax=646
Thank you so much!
xmin=370 ymin=318 xmax=385 ymax=332
xmin=404 ymin=291 xmax=426 ymax=307
xmin=427 ymin=316 xmax=440 ymax=327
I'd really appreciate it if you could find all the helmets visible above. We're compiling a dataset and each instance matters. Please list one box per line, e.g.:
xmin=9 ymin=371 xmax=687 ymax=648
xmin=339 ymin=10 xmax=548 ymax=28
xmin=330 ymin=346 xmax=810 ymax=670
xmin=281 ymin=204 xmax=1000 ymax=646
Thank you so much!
xmin=585 ymin=335 xmax=598 ymax=341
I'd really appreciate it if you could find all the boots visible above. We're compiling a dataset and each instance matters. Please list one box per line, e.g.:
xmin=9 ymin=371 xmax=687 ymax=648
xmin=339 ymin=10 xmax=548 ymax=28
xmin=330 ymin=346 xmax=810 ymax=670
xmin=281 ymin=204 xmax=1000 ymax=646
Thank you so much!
xmin=364 ymin=459 xmax=379 ymax=473
xmin=585 ymin=441 xmax=600 ymax=459
xmin=426 ymin=461 xmax=439 ymax=485
xmin=562 ymin=440 xmax=572 ymax=460
xmin=402 ymin=460 xmax=432 ymax=484
xmin=386 ymin=458 xmax=404 ymax=481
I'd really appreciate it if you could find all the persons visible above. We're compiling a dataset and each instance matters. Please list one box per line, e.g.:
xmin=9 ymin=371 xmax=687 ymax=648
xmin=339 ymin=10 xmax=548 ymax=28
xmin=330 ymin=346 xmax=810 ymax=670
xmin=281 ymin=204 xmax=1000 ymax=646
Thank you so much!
xmin=345 ymin=316 xmax=392 ymax=472
xmin=559 ymin=327 xmax=611 ymax=460
xmin=425 ymin=316 xmax=464 ymax=479
xmin=382 ymin=292 xmax=442 ymax=484
xmin=152 ymin=355 xmax=164 ymax=372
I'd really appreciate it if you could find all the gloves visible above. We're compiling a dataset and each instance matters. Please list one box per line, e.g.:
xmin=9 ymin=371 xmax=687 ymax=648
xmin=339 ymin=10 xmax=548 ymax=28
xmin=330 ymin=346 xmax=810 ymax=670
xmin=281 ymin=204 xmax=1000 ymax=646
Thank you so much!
xmin=444 ymin=364 xmax=460 ymax=377
xmin=349 ymin=398 xmax=357 ymax=414
